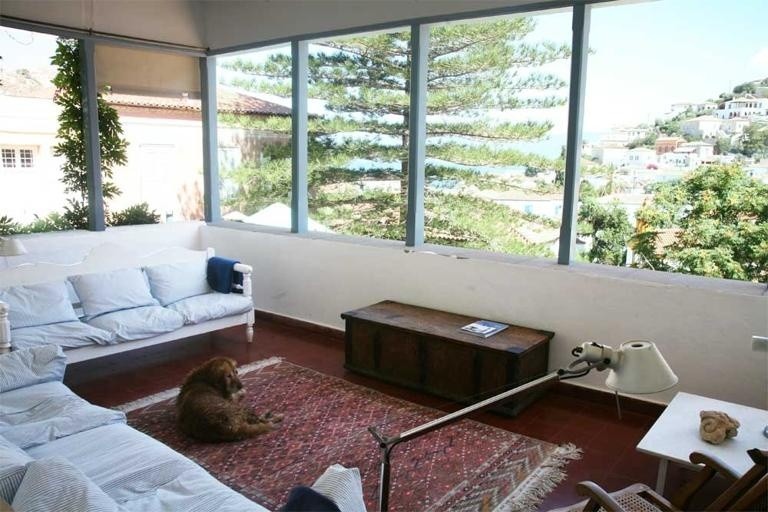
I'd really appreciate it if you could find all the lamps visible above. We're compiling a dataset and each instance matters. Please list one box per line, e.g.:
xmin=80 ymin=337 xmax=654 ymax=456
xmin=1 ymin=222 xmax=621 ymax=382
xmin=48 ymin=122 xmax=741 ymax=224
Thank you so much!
xmin=369 ymin=340 xmax=678 ymax=512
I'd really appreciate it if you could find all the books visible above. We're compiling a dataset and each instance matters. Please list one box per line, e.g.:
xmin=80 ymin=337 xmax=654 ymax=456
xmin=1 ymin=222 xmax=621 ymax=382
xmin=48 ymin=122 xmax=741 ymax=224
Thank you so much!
xmin=456 ymin=319 xmax=509 ymax=339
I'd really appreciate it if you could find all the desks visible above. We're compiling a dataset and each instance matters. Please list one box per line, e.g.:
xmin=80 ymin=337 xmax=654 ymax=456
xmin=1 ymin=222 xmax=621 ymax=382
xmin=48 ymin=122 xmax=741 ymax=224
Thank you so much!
xmin=635 ymin=391 xmax=768 ymax=497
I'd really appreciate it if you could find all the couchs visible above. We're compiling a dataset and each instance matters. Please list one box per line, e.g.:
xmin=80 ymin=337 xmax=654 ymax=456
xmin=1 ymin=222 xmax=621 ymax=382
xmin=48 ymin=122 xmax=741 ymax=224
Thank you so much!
xmin=0 ymin=221 xmax=272 ymax=512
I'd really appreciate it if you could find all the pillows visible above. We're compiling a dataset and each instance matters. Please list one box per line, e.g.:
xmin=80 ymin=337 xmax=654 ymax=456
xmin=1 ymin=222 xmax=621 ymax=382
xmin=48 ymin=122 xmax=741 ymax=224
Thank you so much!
xmin=0 ymin=280 xmax=78 ymax=330
xmin=140 ymin=261 xmax=214 ymax=307
xmin=66 ymin=269 xmax=160 ymax=321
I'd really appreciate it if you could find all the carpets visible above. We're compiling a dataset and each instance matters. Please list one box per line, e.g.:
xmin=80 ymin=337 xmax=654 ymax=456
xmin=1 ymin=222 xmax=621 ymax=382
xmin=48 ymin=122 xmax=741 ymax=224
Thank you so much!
xmin=108 ymin=355 xmax=584 ymax=512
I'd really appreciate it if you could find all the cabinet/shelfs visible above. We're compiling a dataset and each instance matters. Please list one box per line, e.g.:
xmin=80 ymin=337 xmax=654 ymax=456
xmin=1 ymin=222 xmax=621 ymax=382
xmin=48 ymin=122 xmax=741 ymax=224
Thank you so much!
xmin=340 ymin=300 xmax=554 ymax=419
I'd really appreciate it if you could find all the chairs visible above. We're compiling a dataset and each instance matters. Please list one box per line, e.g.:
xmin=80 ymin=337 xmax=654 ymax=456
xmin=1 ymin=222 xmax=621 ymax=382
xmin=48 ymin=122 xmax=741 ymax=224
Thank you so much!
xmin=546 ymin=449 xmax=768 ymax=512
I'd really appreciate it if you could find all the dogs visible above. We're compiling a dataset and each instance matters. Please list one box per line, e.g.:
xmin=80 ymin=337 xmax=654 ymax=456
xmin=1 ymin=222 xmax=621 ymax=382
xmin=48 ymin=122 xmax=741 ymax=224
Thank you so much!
xmin=175 ymin=356 xmax=285 ymax=441
